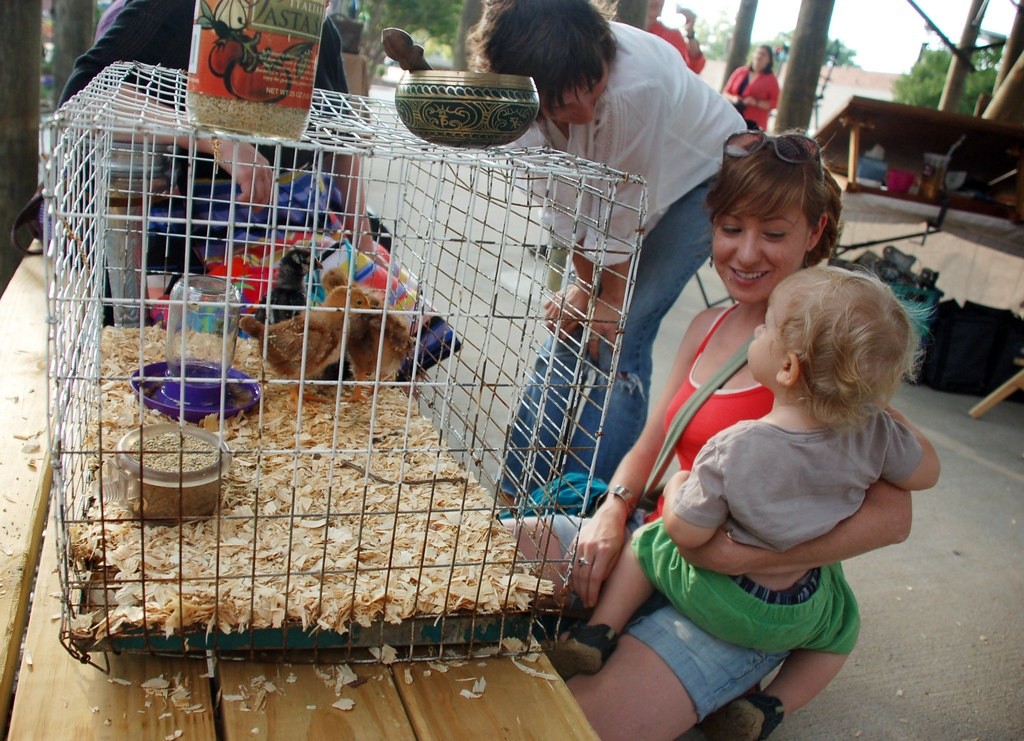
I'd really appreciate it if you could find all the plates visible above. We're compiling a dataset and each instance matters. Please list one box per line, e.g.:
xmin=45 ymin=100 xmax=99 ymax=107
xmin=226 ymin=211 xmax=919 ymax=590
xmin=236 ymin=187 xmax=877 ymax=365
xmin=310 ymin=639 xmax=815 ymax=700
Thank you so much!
xmin=128 ymin=362 xmax=261 ymax=423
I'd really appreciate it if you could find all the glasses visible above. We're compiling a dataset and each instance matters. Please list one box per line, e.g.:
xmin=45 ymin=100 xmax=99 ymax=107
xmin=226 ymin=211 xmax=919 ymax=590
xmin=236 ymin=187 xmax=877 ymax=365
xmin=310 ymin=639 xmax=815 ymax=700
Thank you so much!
xmin=723 ymin=130 xmax=822 ymax=184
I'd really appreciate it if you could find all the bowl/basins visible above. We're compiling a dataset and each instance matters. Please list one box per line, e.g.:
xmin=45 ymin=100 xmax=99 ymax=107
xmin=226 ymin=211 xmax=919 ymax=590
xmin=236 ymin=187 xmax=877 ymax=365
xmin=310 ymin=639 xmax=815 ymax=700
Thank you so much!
xmin=395 ymin=70 xmax=541 ymax=150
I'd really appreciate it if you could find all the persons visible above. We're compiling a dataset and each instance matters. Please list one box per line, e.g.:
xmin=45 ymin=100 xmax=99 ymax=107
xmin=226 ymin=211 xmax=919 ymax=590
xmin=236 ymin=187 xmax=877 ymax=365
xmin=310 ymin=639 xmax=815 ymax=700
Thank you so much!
xmin=497 ymin=128 xmax=911 ymax=741
xmin=471 ymin=0 xmax=752 ymax=516
xmin=40 ymin=0 xmax=461 ymax=382
xmin=539 ymin=266 xmax=940 ymax=741
xmin=724 ymin=45 xmax=778 ymax=132
xmin=644 ymin=0 xmax=706 ymax=75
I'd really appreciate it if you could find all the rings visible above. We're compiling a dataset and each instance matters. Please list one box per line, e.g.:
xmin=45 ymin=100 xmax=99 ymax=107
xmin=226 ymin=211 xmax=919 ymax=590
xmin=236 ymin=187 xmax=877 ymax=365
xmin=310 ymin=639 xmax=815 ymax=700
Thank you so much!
xmin=578 ymin=558 xmax=591 ymax=566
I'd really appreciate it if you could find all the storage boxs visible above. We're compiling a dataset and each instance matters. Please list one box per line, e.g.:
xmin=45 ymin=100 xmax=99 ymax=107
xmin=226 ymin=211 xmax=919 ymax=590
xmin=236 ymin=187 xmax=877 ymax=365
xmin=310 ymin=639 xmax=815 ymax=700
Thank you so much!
xmin=865 ymin=260 xmax=944 ymax=339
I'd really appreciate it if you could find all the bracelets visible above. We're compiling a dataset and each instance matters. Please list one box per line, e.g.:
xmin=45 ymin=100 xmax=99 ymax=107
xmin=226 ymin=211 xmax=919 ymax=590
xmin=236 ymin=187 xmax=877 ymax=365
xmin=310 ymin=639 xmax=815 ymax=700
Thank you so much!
xmin=596 ymin=486 xmax=637 ymax=520
xmin=687 ymin=33 xmax=694 ymax=39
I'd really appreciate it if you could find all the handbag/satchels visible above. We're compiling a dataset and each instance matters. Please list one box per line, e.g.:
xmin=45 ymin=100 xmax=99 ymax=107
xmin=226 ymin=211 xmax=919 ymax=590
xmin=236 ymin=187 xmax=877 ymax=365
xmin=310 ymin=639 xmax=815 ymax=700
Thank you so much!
xmin=922 ymin=298 xmax=1024 ymax=404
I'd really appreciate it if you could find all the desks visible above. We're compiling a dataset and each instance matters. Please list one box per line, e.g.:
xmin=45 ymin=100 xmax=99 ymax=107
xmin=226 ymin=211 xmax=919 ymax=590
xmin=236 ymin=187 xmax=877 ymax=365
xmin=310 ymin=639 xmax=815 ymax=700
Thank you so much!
xmin=827 ymin=172 xmax=1024 ymax=280
xmin=0 ymin=239 xmax=602 ymax=741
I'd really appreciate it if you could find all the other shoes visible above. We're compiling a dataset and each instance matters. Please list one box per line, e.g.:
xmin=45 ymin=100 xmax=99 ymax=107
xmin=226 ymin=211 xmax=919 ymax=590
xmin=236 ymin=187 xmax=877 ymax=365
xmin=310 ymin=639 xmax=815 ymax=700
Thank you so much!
xmin=548 ymin=623 xmax=618 ymax=679
xmin=700 ymin=692 xmax=785 ymax=741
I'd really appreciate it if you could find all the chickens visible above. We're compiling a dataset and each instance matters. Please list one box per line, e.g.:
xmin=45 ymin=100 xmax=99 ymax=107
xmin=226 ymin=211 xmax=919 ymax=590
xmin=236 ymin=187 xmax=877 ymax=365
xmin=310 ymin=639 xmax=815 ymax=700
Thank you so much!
xmin=237 ymin=248 xmax=414 ymax=410
